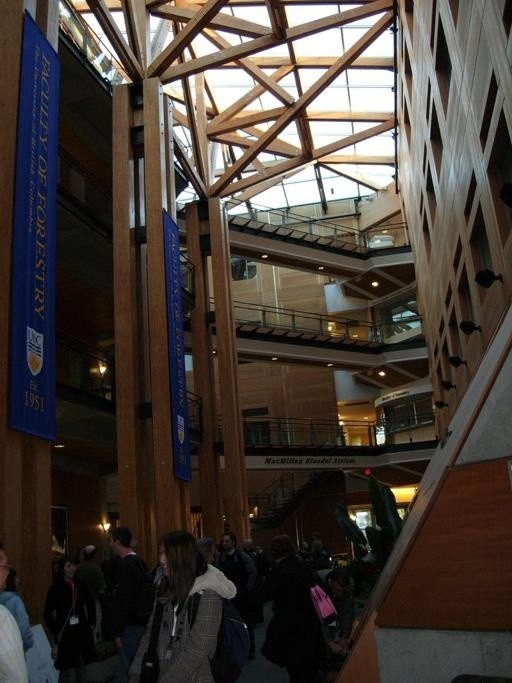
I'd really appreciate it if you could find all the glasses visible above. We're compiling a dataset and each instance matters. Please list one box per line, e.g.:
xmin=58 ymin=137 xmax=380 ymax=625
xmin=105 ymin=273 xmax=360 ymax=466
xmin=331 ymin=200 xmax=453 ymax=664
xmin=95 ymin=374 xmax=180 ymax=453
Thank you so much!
xmin=0 ymin=564 xmax=14 ymax=573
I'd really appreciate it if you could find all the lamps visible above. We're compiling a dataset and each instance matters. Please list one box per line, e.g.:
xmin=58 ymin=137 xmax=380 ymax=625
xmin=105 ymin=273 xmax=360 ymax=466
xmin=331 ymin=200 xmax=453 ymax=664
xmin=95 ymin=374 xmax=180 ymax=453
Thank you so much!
xmin=435 ymin=401 xmax=448 ymax=409
xmin=440 ymin=380 xmax=456 ymax=391
xmin=476 ymin=269 xmax=502 ymax=288
xmin=450 ymin=357 xmax=466 ymax=368
xmin=460 ymin=321 xmax=480 ymax=335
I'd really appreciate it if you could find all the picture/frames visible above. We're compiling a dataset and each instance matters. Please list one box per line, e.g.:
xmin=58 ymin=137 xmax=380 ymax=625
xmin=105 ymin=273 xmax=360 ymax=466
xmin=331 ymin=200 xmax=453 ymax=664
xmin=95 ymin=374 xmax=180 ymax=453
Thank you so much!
xmin=52 ymin=504 xmax=70 ymax=558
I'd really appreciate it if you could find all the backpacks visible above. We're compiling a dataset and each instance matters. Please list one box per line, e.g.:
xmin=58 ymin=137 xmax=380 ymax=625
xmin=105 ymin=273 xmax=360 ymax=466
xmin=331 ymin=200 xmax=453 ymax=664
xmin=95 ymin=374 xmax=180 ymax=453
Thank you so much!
xmin=209 ymin=598 xmax=251 ymax=683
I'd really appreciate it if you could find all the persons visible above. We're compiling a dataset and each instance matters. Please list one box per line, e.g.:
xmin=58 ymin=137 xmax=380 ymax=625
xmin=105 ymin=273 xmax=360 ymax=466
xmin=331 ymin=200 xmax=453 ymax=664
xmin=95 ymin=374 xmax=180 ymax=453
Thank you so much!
xmin=1 ymin=528 xmax=356 ymax=682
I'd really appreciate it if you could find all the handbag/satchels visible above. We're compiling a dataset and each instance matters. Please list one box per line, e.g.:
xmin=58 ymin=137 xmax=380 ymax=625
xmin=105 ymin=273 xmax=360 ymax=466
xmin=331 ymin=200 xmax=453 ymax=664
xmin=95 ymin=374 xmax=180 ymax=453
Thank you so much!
xmin=308 ymin=584 xmax=339 ymax=627
xmin=50 ymin=640 xmax=60 ymax=663
xmin=137 ymin=651 xmax=162 ymax=683
